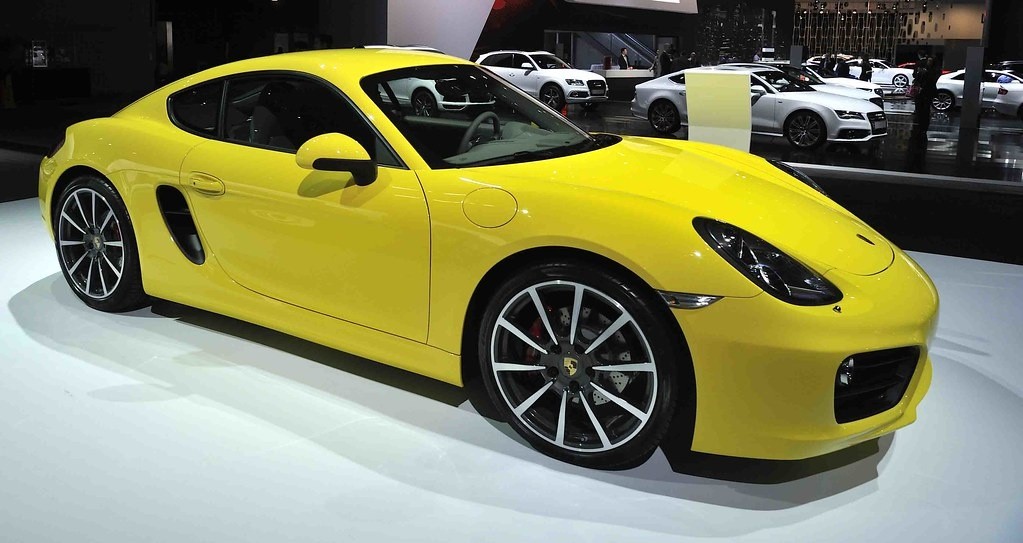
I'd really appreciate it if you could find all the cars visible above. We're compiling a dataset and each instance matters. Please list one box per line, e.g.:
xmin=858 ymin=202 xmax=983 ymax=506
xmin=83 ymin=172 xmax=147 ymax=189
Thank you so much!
xmin=628 ymin=66 xmax=888 ymax=150
xmin=897 ymin=63 xmax=951 ymax=75
xmin=722 ymin=62 xmax=885 ymax=112
xmin=38 ymin=47 xmax=939 ymax=471
xmin=833 ymin=59 xmax=915 ymax=88
xmin=771 ymin=61 xmax=884 ymax=97
xmin=993 ymin=83 xmax=1023 ymax=121
xmin=988 ymin=59 xmax=1023 ymax=71
xmin=932 ymin=69 xmax=1023 ymax=113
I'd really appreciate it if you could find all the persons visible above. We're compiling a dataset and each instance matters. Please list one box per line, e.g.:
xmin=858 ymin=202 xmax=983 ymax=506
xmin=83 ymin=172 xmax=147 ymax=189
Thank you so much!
xmin=753 ymin=50 xmax=760 ymax=61
xmin=836 ymin=59 xmax=849 ymax=77
xmin=648 ymin=43 xmax=698 ymax=79
xmin=618 ymin=48 xmax=633 ymax=70
xmin=859 ymin=58 xmax=872 ymax=81
xmin=818 ymin=51 xmax=835 ymax=76
xmin=913 ymin=50 xmax=940 ymax=134
xmin=295 ymin=35 xmax=333 ymax=51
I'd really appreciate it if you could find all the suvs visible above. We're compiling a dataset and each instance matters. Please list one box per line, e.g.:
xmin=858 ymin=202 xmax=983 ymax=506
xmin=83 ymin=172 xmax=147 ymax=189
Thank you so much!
xmin=352 ymin=45 xmax=496 ymax=118
xmin=806 ymin=56 xmax=824 ymax=63
xmin=474 ymin=50 xmax=608 ymax=112
